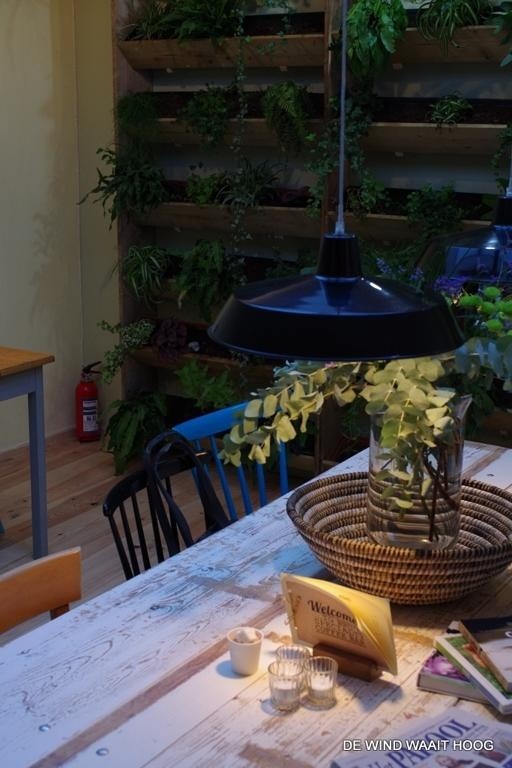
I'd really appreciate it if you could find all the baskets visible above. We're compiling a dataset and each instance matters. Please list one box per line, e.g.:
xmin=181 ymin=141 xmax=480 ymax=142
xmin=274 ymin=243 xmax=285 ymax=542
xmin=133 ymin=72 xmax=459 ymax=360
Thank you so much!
xmin=285 ymin=473 xmax=511 ymax=605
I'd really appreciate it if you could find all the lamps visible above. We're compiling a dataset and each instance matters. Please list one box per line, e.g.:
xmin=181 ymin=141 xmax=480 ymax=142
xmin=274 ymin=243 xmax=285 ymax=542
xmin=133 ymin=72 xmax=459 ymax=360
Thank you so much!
xmin=207 ymin=1 xmax=466 ymax=364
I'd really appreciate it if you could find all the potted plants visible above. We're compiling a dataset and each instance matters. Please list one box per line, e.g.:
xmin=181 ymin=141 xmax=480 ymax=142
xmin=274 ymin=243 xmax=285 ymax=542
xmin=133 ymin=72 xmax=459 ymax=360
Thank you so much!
xmin=218 ymin=353 xmax=470 ymax=549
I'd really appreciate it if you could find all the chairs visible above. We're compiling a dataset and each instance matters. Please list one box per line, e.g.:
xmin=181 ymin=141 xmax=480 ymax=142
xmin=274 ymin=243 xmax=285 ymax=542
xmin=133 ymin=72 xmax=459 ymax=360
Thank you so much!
xmin=3 ymin=547 xmax=84 ymax=644
xmin=172 ymin=402 xmax=289 ymax=520
xmin=102 ymin=465 xmax=178 ymax=582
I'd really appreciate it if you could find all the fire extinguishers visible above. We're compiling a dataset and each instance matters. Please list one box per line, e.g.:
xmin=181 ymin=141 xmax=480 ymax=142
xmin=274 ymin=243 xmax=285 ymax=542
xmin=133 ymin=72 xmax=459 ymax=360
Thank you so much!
xmin=71 ymin=361 xmax=104 ymax=442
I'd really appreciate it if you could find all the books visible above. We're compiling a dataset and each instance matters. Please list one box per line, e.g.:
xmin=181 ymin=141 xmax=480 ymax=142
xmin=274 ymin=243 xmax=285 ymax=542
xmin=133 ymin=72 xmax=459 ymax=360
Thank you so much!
xmin=417 ymin=614 xmax=512 ymax=716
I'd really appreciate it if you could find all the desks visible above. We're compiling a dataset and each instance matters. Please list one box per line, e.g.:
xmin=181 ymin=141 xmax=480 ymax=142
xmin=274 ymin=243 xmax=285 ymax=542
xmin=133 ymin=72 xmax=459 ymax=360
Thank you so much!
xmin=2 ymin=441 xmax=509 ymax=766
xmin=0 ymin=348 xmax=58 ymax=555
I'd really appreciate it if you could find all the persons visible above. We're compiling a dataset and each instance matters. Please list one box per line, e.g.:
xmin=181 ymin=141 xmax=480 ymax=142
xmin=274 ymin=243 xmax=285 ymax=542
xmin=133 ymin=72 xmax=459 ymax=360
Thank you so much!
xmin=436 ymin=755 xmax=474 ymax=767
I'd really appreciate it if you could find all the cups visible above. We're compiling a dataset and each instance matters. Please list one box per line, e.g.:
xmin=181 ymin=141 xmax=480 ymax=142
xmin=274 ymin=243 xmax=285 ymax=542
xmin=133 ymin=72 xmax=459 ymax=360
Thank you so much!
xmin=267 ymin=659 xmax=303 ymax=711
xmin=305 ymin=656 xmax=337 ymax=703
xmin=277 ymin=645 xmax=310 ymax=692
xmin=226 ymin=626 xmax=264 ymax=676
